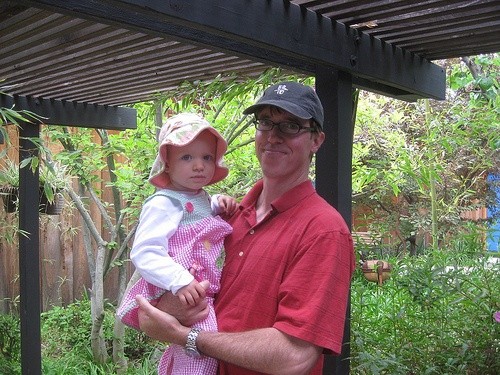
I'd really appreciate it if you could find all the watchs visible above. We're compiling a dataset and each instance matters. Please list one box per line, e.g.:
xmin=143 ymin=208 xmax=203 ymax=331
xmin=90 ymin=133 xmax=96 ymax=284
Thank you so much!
xmin=185 ymin=328 xmax=204 ymax=358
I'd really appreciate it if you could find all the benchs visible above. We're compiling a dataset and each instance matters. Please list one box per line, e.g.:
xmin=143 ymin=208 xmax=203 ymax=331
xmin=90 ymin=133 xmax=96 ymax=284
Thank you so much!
xmin=351 ymin=232 xmax=384 ymax=259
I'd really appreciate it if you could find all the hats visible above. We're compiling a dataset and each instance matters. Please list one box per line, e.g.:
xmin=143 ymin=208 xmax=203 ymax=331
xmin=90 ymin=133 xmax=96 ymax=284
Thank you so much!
xmin=148 ymin=112 xmax=229 ymax=187
xmin=242 ymin=81 xmax=326 ymax=130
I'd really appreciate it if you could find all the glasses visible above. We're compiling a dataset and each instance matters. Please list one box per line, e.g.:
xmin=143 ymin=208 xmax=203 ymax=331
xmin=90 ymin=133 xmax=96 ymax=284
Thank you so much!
xmin=253 ymin=117 xmax=317 ymax=134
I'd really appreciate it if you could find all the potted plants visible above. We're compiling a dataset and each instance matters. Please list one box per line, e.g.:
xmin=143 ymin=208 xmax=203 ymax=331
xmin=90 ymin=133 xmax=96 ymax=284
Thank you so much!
xmin=38 ymin=160 xmax=82 ymax=286
xmin=0 ymin=155 xmax=32 ymax=307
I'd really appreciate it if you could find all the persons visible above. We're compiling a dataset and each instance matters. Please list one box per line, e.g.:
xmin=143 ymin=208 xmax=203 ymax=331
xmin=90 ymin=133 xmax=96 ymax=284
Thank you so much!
xmin=136 ymin=81 xmax=354 ymax=375
xmin=117 ymin=113 xmax=238 ymax=375
